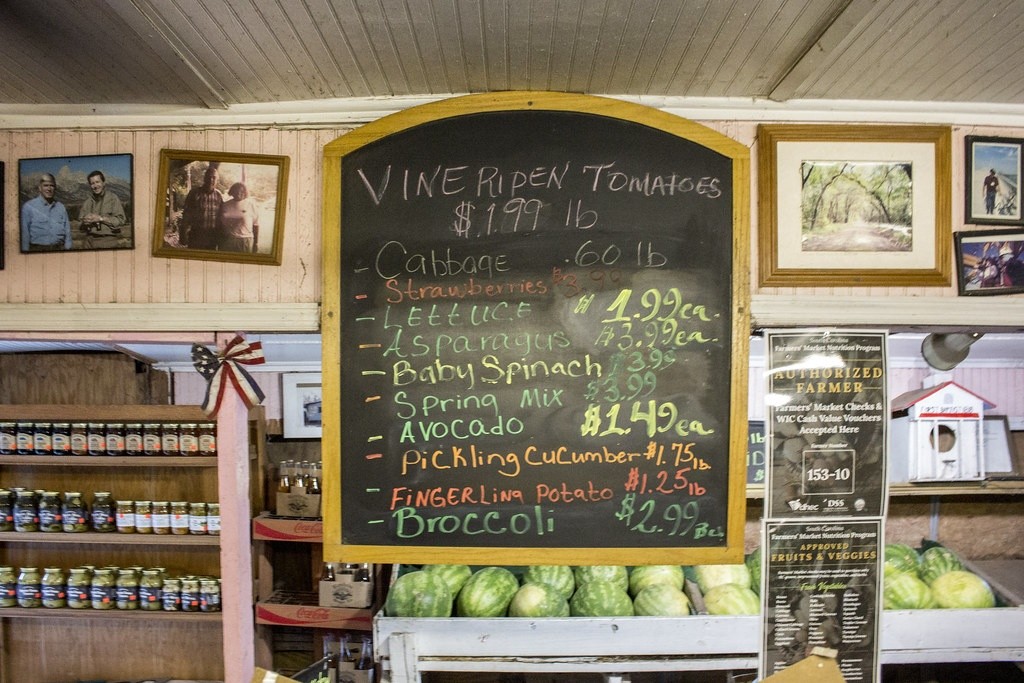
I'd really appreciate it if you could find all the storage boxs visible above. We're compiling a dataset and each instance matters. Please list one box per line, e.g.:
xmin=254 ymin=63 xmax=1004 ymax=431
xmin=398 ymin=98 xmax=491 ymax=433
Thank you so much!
xmin=323 ymin=642 xmax=374 ymax=683
xmin=275 ymin=491 xmax=321 ymax=518
xmin=318 ymin=580 xmax=374 ymax=609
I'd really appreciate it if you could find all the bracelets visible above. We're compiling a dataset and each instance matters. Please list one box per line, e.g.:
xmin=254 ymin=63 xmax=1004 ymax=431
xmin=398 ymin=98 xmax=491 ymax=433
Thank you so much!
xmin=99 ymin=215 xmax=103 ymax=222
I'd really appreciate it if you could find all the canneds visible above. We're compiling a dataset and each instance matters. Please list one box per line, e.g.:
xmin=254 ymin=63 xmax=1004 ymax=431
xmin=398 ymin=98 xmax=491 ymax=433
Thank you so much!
xmin=0 ymin=422 xmax=218 ymax=456
xmin=0 ymin=487 xmax=88 ymax=533
xmin=163 ymin=575 xmax=222 ymax=612
xmin=91 ymin=491 xmax=116 ymax=532
xmin=117 ymin=500 xmax=221 ymax=536
xmin=0 ymin=563 xmax=167 ymax=610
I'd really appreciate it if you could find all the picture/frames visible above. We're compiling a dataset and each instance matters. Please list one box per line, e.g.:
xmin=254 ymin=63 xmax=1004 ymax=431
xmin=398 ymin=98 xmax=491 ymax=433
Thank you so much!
xmin=757 ymin=123 xmax=955 ymax=288
xmin=18 ymin=153 xmax=135 ymax=253
xmin=281 ymin=371 xmax=320 ymax=439
xmin=963 ymin=135 xmax=1024 ymax=226
xmin=953 ymin=229 xmax=1024 ymax=297
xmin=151 ymin=148 xmax=290 ymax=266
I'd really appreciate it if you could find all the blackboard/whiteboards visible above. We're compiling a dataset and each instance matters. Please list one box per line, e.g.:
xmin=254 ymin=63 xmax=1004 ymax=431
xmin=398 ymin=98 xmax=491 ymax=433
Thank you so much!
xmin=322 ymin=91 xmax=751 ymax=565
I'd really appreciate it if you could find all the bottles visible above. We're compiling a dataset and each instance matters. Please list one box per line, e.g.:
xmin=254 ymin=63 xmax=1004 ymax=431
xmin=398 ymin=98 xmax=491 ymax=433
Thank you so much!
xmin=322 ymin=631 xmax=373 ymax=678
xmin=278 ymin=459 xmax=322 ymax=494
xmin=321 ymin=561 xmax=335 ymax=582
xmin=0 ymin=421 xmax=217 ymax=457
xmin=0 ymin=563 xmax=222 ymax=614
xmin=0 ymin=486 xmax=220 ymax=537
xmin=338 ymin=563 xmax=370 ymax=582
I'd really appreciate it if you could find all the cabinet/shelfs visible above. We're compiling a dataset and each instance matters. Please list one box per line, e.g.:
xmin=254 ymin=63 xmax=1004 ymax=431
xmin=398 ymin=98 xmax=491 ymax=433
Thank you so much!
xmin=253 ymin=510 xmax=382 ymax=630
xmin=0 ymin=405 xmax=265 ymax=682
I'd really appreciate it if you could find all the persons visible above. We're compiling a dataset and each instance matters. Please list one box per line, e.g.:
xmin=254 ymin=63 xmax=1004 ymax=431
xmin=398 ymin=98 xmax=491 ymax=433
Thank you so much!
xmin=19 ymin=172 xmax=72 ymax=253
xmin=982 ymin=168 xmax=999 ymax=215
xmin=218 ymin=183 xmax=260 ymax=257
xmin=176 ymin=166 xmax=223 ymax=252
xmin=77 ymin=171 xmax=127 ymax=251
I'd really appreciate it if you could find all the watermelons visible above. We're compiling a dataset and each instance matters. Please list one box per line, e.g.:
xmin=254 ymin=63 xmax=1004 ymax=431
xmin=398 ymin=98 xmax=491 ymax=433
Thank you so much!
xmin=386 ymin=547 xmax=761 ymax=617
xmin=883 ymin=542 xmax=996 ymax=609
